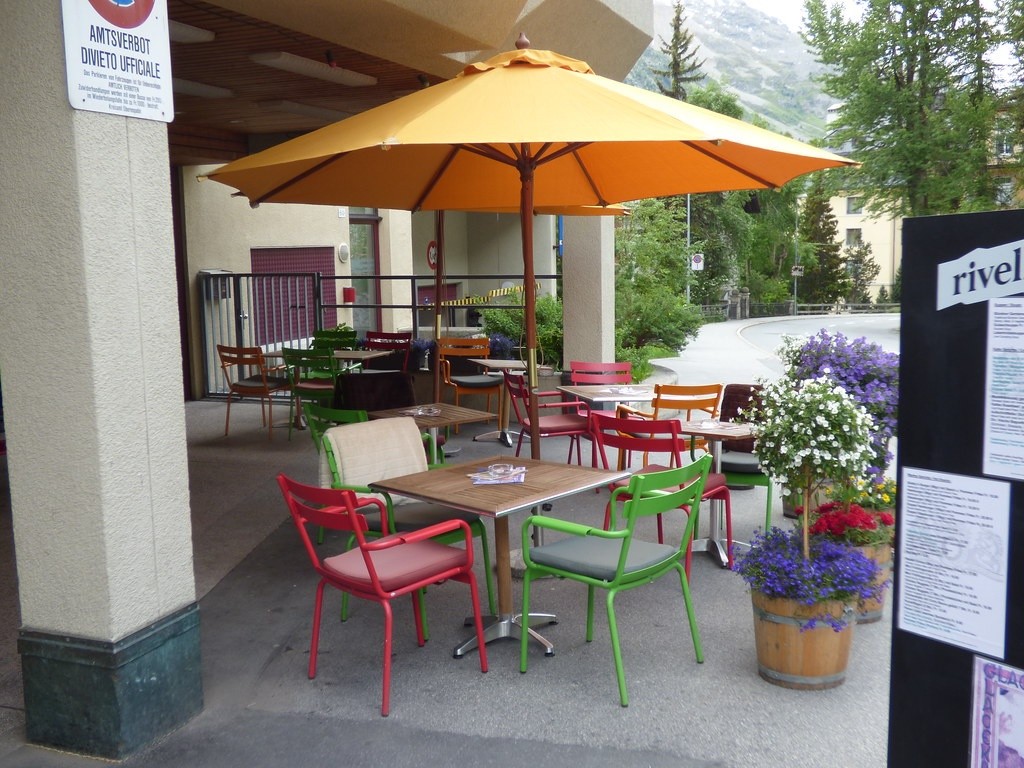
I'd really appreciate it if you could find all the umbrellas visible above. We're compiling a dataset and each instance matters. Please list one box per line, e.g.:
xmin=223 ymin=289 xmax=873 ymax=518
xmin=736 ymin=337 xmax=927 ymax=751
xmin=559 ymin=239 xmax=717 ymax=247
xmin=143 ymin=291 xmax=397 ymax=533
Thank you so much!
xmin=430 ymin=202 xmax=632 ymax=438
xmin=195 ymin=31 xmax=864 ymax=544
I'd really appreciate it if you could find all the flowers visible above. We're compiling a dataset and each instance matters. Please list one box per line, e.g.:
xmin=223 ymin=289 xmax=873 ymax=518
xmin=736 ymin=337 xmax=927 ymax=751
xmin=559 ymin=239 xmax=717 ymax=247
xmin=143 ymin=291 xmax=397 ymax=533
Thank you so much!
xmin=734 ymin=330 xmax=899 ymax=630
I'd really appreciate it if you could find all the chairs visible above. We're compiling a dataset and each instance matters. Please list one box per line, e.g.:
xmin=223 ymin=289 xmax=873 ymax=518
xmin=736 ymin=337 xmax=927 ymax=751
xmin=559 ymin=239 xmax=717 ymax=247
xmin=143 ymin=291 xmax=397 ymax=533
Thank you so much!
xmin=216 ymin=331 xmax=798 ymax=717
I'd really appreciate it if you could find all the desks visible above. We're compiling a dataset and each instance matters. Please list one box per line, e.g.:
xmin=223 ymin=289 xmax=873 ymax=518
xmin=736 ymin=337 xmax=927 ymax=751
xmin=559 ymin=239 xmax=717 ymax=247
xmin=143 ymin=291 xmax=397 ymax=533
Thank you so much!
xmin=468 ymin=358 xmax=555 ymax=448
xmin=367 ymin=455 xmax=633 ymax=659
xmin=555 ymin=384 xmax=693 ymax=471
xmin=368 ymin=402 xmax=498 ymax=468
xmin=672 ymin=421 xmax=761 ymax=569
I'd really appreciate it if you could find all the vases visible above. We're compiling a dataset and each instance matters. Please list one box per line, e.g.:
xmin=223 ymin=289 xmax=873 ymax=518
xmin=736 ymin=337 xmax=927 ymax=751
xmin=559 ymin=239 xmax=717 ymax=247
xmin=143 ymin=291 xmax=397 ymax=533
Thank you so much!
xmin=748 ymin=582 xmax=850 ymax=689
xmin=842 ymin=540 xmax=891 ymax=622
